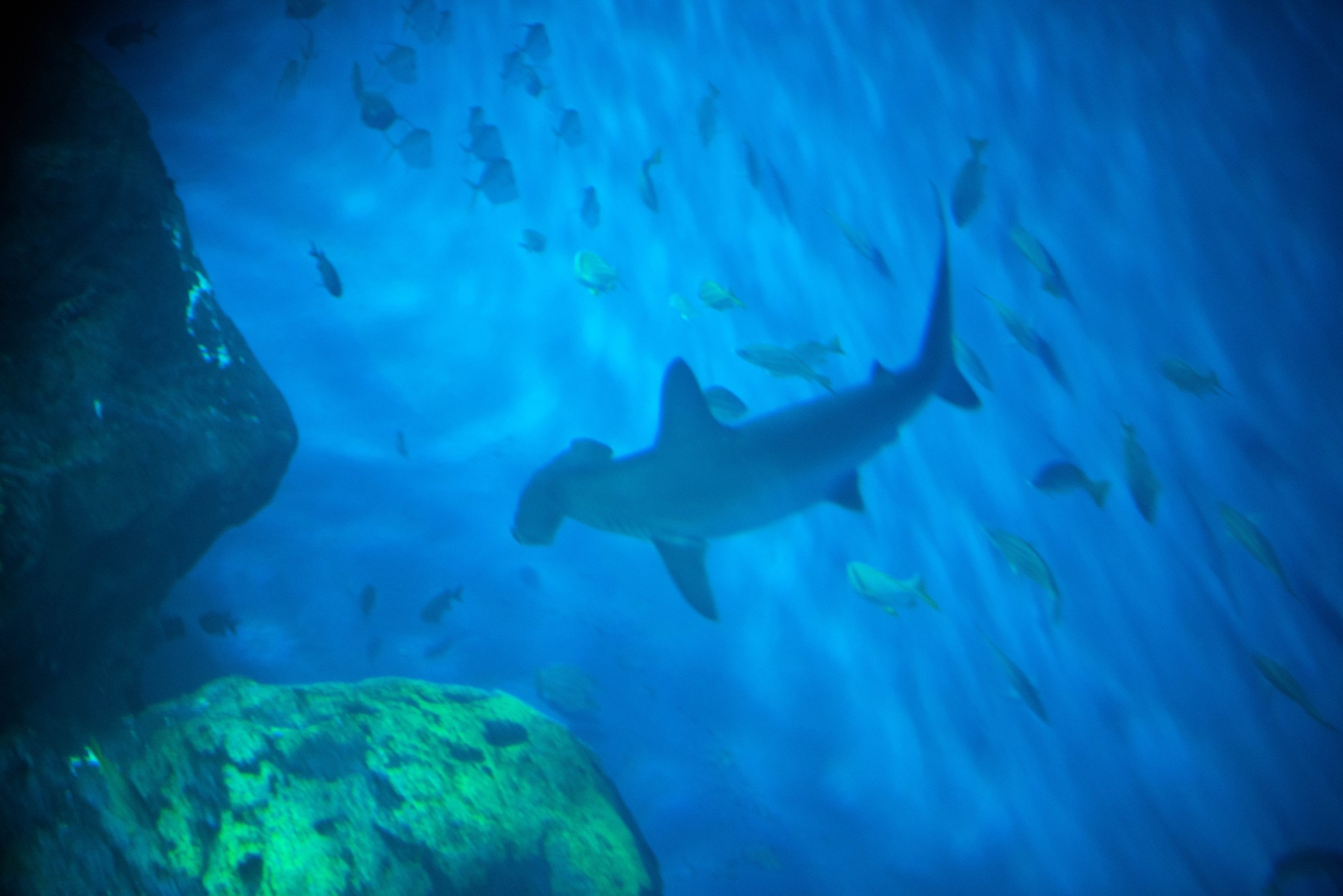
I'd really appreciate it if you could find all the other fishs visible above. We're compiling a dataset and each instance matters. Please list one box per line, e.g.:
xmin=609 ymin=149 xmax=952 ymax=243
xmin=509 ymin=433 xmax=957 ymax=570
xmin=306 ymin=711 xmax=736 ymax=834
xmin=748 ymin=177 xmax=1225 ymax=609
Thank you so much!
xmin=848 ymin=136 xmax=1343 ymax=742
xmin=176 ymin=536 xmax=479 ymax=679
xmin=274 ymin=0 xmax=899 ymax=395
